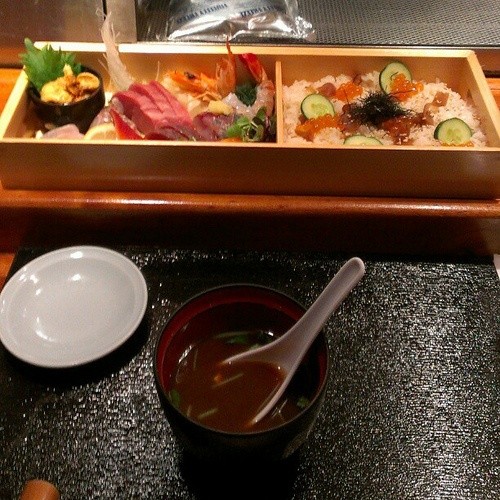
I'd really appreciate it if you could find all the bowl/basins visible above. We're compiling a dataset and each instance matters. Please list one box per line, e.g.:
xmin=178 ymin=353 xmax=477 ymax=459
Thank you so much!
xmin=151 ymin=283 xmax=333 ymax=467
xmin=28 ymin=63 xmax=106 ymax=125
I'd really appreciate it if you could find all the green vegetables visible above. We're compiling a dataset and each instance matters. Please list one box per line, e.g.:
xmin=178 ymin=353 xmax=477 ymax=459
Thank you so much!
xmin=18 ymin=36 xmax=82 ymax=96
xmin=228 ymin=104 xmax=276 ymax=143
xmin=169 ymin=319 xmax=312 ymax=432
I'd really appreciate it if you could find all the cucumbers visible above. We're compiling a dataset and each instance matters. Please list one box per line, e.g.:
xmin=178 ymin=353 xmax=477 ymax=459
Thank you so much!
xmin=300 ymin=93 xmax=335 ymax=119
xmin=343 ymin=135 xmax=383 ymax=146
xmin=434 ymin=117 xmax=473 ymax=141
xmin=379 ymin=61 xmax=413 ymax=96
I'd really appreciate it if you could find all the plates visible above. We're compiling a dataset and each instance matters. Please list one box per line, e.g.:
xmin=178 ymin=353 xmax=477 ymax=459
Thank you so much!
xmin=0 ymin=245 xmax=150 ymax=369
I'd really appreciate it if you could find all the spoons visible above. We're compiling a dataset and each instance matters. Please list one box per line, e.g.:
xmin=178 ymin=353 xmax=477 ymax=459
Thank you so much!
xmin=214 ymin=256 xmax=368 ymax=428
